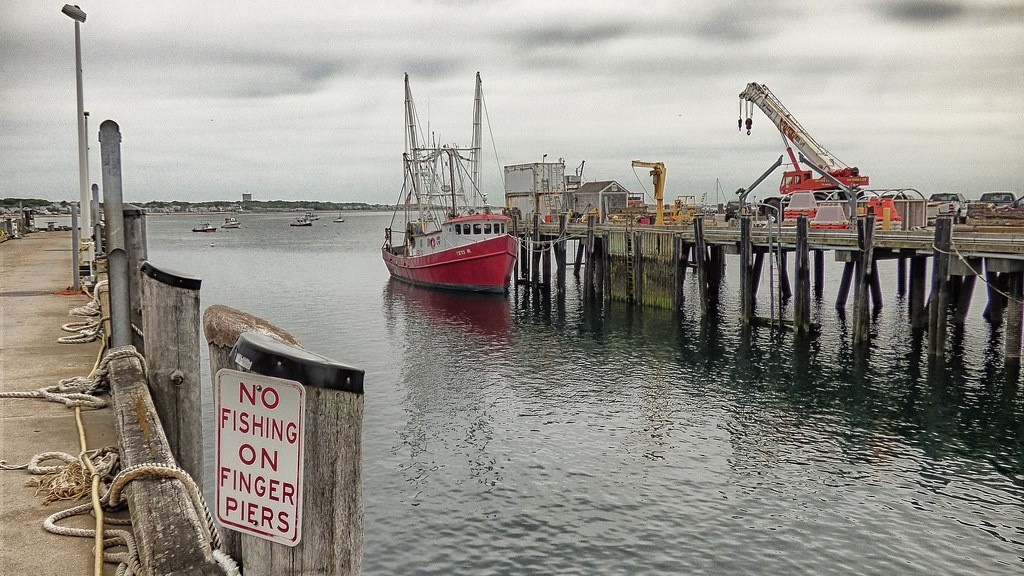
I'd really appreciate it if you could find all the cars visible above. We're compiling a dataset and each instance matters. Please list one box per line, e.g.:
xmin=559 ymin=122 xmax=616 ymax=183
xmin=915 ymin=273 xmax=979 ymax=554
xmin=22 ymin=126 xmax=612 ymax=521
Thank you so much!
xmin=981 ymin=190 xmax=1018 ymax=208
xmin=926 ymin=192 xmax=972 ymax=225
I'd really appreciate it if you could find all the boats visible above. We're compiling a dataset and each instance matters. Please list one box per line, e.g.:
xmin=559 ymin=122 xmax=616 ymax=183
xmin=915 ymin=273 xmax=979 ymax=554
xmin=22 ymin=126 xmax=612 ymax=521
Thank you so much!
xmin=192 ymin=222 xmax=217 ymax=233
xmin=290 ymin=211 xmax=320 ymax=226
xmin=220 ymin=218 xmax=241 ymax=228
xmin=333 ymin=210 xmax=345 ymax=223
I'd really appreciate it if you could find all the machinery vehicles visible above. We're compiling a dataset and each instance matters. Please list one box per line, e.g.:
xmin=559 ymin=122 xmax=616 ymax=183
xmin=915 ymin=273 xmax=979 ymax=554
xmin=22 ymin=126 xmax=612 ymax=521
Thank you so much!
xmin=725 ymin=154 xmax=784 ymax=222
xmin=737 ymin=82 xmax=902 ymax=233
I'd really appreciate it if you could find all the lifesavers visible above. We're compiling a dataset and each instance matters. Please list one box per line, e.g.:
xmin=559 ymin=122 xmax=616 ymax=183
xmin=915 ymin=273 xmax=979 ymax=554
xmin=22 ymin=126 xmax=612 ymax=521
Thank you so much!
xmin=430 ymin=238 xmax=436 ymax=250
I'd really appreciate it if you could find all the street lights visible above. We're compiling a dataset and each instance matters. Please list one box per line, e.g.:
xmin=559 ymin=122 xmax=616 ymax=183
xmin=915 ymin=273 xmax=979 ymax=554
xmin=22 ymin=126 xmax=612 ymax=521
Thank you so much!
xmin=542 ymin=154 xmax=547 ymax=224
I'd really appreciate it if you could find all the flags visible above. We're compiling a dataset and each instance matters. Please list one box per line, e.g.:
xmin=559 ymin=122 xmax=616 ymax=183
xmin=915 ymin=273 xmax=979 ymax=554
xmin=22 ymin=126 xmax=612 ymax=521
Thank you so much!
xmin=406 ymin=191 xmax=411 ymax=205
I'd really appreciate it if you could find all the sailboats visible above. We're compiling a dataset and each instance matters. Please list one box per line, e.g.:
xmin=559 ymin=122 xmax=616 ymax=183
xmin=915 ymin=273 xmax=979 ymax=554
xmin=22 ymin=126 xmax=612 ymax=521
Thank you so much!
xmin=380 ymin=70 xmax=521 ymax=293
